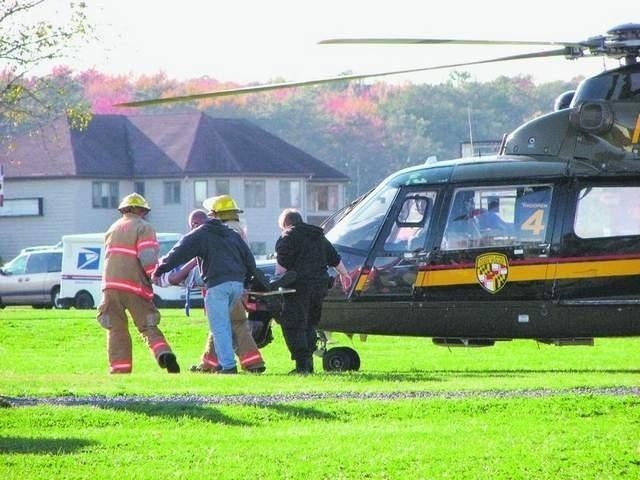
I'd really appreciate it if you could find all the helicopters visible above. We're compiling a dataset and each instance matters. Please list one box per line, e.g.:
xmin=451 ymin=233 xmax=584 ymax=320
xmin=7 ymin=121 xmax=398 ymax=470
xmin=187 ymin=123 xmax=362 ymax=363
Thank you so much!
xmin=109 ymin=18 xmax=639 ymax=374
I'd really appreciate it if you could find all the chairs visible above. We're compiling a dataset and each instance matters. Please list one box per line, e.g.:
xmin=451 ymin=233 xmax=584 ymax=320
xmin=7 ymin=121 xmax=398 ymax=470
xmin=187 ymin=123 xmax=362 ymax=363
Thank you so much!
xmin=444 ymin=189 xmax=476 ymax=236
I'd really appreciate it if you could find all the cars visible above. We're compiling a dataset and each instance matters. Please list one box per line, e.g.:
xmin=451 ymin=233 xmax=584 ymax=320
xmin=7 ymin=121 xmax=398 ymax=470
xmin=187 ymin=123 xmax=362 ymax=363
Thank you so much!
xmin=0 ymin=241 xmax=66 ymax=310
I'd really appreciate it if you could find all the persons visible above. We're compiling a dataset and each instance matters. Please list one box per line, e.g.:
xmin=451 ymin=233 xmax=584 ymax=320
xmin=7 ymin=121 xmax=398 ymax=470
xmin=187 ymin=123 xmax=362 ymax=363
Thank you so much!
xmin=274 ymin=209 xmax=351 ymax=375
xmin=151 ymin=210 xmax=257 ymax=374
xmin=100 ymin=193 xmax=181 ymax=373
xmin=155 ymin=257 xmax=297 ymax=292
xmin=190 ymin=195 xmax=266 ymax=373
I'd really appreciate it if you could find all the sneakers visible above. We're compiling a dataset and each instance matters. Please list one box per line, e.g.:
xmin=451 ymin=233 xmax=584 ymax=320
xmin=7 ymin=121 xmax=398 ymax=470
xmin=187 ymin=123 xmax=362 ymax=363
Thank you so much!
xmin=271 ymin=270 xmax=297 ymax=291
xmin=191 ymin=363 xmax=265 ymax=374
xmin=162 ymin=353 xmax=180 ymax=373
xmin=254 ymin=271 xmax=272 ymax=290
xmin=289 ymin=369 xmax=312 ymax=375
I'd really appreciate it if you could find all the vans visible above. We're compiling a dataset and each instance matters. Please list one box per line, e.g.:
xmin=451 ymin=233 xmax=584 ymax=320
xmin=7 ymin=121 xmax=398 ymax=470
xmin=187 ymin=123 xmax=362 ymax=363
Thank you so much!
xmin=59 ymin=228 xmax=208 ymax=309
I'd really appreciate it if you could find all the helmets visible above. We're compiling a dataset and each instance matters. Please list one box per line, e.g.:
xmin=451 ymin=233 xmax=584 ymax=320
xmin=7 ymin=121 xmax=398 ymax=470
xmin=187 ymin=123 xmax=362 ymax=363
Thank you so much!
xmin=118 ymin=193 xmax=150 ymax=211
xmin=203 ymin=195 xmax=242 ymax=221
